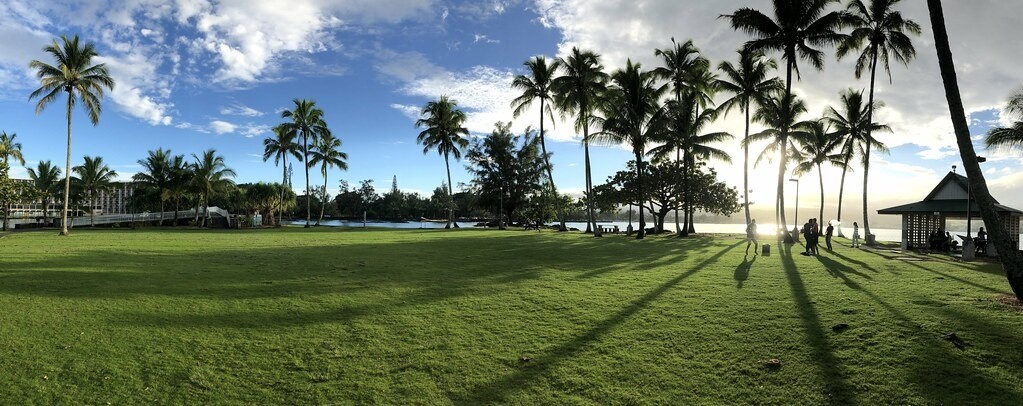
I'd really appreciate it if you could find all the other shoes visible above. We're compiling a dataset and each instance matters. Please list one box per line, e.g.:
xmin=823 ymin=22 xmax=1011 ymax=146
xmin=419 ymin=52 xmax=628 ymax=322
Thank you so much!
xmin=754 ymin=251 xmax=758 ymax=255
xmin=855 ymin=246 xmax=859 ymax=248
xmin=746 ymin=249 xmax=748 ymax=255
xmin=850 ymin=245 xmax=854 ymax=248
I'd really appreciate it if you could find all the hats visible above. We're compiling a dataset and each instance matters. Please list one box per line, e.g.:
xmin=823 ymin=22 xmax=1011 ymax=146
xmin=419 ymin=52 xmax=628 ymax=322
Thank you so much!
xmin=812 ymin=218 xmax=817 ymax=221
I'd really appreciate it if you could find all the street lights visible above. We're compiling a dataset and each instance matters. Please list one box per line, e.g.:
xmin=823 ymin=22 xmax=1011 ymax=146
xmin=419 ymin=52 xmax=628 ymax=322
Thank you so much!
xmin=789 ymin=178 xmax=799 ymax=239
xmin=961 ymin=156 xmax=987 ymax=259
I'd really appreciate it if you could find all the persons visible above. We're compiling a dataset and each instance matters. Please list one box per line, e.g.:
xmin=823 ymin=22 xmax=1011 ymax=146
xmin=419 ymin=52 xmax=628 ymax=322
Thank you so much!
xmin=976 ymin=227 xmax=993 ymax=254
xmin=504 ymin=216 xmax=509 ymax=228
xmin=825 ymin=221 xmax=834 ymax=252
xmin=525 ymin=217 xmax=540 ymax=231
xmin=746 ymin=219 xmax=759 ymax=255
xmin=929 ymin=228 xmax=953 ymax=252
xmin=801 ymin=218 xmax=820 ymax=255
xmin=850 ymin=222 xmax=859 ymax=248
